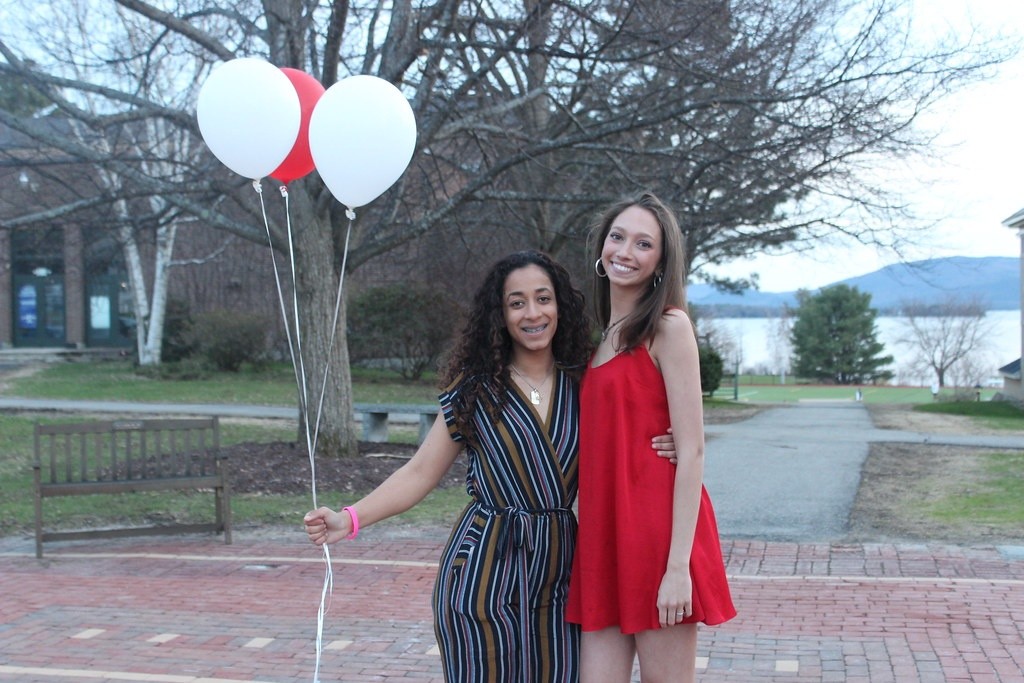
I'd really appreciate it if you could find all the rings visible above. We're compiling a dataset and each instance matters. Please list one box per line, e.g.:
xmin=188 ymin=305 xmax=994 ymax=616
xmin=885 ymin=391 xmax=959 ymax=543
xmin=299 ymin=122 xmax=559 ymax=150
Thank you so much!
xmin=676 ymin=612 xmax=684 ymax=615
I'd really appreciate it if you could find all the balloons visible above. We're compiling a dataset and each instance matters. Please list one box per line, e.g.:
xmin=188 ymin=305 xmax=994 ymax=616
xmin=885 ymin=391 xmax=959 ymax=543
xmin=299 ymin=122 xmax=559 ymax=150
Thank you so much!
xmin=197 ymin=57 xmax=301 ymax=192
xmin=308 ymin=75 xmax=417 ymax=220
xmin=267 ymin=67 xmax=326 ymax=196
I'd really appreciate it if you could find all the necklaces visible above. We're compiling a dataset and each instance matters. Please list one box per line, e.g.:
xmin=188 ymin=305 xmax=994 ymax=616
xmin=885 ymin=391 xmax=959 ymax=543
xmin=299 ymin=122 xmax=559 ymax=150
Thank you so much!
xmin=511 ymin=361 xmax=554 ymax=405
xmin=601 ymin=313 xmax=631 ymax=341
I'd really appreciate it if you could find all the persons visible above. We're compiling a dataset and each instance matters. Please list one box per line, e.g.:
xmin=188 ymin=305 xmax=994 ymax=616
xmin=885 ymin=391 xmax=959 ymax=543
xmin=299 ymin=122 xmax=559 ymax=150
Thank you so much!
xmin=305 ymin=248 xmax=678 ymax=683
xmin=564 ymin=190 xmax=738 ymax=683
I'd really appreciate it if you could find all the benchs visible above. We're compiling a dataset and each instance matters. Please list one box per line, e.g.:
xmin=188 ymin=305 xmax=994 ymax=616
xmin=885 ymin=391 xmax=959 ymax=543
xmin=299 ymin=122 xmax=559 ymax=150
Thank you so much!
xmin=28 ymin=411 xmax=234 ymax=558
xmin=351 ymin=401 xmax=441 ymax=446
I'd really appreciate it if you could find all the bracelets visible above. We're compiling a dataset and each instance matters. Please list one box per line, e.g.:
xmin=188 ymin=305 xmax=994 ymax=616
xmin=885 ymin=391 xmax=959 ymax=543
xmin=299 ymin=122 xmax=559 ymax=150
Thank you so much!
xmin=342 ymin=506 xmax=359 ymax=541
